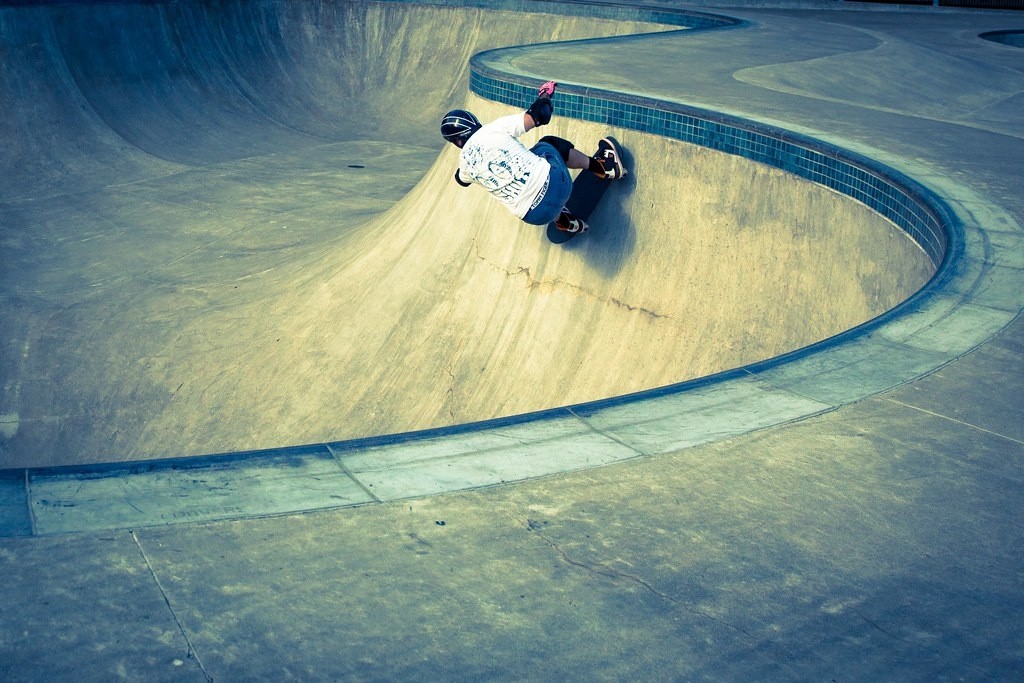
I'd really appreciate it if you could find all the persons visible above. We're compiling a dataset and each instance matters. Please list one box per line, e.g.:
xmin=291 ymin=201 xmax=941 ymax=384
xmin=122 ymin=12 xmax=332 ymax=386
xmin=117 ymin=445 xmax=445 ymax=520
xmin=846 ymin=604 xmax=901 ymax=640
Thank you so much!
xmin=441 ymin=81 xmax=623 ymax=232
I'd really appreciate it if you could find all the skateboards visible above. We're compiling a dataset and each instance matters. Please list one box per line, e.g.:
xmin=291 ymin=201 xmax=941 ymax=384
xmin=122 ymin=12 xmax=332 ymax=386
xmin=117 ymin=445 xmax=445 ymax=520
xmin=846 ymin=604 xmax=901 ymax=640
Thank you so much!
xmin=546 ymin=135 xmax=628 ymax=245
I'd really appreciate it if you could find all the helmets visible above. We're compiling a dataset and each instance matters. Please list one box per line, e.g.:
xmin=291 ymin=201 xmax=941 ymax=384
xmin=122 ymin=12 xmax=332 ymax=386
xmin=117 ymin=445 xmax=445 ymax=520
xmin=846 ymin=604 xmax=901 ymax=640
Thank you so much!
xmin=440 ymin=109 xmax=483 ymax=142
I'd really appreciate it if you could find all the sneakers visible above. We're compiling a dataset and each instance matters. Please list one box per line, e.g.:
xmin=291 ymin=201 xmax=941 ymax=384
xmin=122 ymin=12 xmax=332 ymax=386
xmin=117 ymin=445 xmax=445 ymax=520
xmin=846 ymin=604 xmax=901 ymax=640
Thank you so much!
xmin=591 ymin=138 xmax=625 ymax=181
xmin=556 ymin=206 xmax=585 ymax=234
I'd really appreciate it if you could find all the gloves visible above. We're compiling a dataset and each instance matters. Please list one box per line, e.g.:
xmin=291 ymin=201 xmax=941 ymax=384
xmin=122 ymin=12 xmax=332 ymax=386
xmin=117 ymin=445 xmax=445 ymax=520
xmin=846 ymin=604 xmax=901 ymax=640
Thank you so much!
xmin=538 ymin=80 xmax=558 ymax=98
